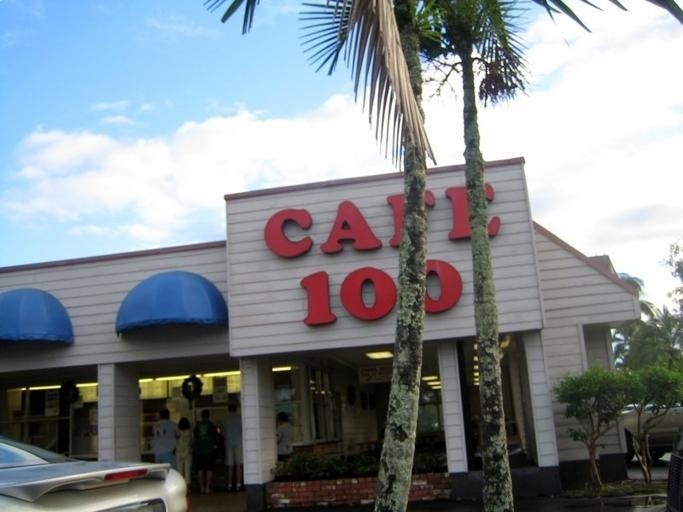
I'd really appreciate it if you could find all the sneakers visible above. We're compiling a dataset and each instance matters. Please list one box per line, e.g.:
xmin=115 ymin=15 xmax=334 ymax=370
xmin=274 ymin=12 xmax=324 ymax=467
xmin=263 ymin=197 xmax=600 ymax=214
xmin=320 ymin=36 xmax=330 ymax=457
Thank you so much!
xmin=226 ymin=483 xmax=241 ymax=492
xmin=200 ymin=488 xmax=212 ymax=495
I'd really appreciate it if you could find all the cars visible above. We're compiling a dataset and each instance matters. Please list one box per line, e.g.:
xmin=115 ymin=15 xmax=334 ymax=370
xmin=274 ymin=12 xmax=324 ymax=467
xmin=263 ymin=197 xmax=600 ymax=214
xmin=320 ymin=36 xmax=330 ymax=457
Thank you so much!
xmin=622 ymin=402 xmax=683 ymax=462
xmin=1 ymin=438 xmax=189 ymax=512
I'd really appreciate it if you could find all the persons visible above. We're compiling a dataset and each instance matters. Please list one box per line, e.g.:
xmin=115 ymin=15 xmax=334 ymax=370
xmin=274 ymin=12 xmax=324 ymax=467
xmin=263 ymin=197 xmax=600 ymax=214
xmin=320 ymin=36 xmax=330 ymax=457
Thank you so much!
xmin=154 ymin=401 xmax=242 ymax=493
xmin=276 ymin=412 xmax=294 ymax=461
